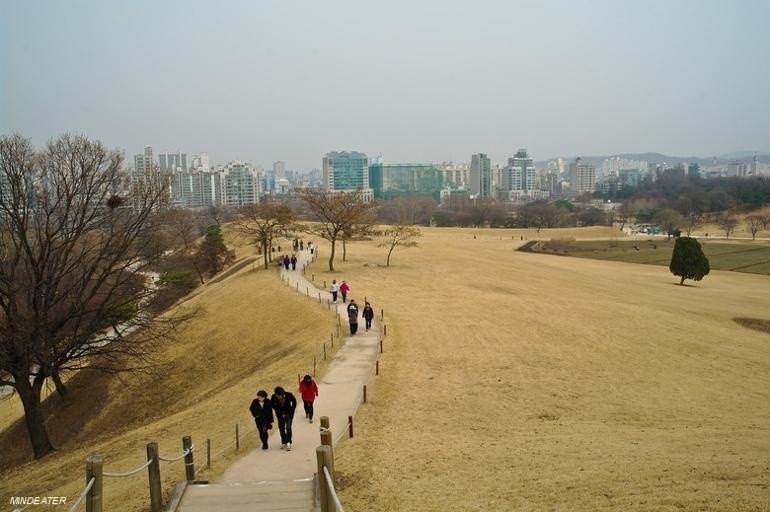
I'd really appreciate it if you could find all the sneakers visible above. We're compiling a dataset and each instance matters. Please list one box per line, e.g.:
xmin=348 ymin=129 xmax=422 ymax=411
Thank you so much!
xmin=280 ymin=442 xmax=291 ymax=452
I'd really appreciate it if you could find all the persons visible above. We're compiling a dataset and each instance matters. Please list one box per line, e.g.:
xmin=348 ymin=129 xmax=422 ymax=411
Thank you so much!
xmin=339 ymin=281 xmax=350 ymax=303
xmin=299 ymin=375 xmax=319 ymax=424
xmin=330 ymin=280 xmax=340 ymax=303
xmin=362 ymin=302 xmax=374 ymax=332
xmin=349 ymin=306 xmax=357 ymax=334
xmin=347 ymin=299 xmax=358 ymax=314
xmin=284 ymin=254 xmax=290 ymax=270
xmin=291 ymin=254 xmax=297 ymax=270
xmin=249 ymin=390 xmax=275 ymax=450
xmin=270 ymin=386 xmax=297 ymax=451
xmin=256 ymin=226 xmax=314 ymax=255
